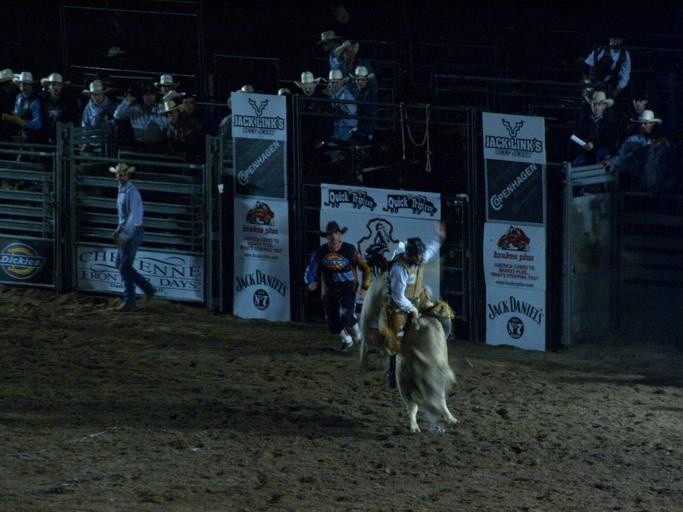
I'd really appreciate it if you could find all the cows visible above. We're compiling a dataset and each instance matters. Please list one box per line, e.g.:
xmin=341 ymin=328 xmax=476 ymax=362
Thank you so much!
xmin=356 ymin=270 xmax=460 ymax=435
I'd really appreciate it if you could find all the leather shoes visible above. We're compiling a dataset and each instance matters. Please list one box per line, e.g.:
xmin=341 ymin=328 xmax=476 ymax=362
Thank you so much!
xmin=113 ymin=302 xmax=137 ymax=312
xmin=386 ymin=367 xmax=397 ymax=389
xmin=143 ymin=287 xmax=156 ymax=302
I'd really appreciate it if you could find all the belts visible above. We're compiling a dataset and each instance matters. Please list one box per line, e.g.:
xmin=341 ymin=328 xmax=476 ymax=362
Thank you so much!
xmin=120 ymin=223 xmax=143 ymax=229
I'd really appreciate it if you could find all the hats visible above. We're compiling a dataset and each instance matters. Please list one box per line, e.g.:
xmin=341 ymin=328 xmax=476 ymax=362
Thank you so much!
xmin=315 ymin=31 xmax=342 ymax=46
xmin=323 ymin=69 xmax=350 ymax=84
xmin=239 ymin=86 xmax=253 ymax=92
xmin=277 ymin=88 xmax=290 ymax=97
xmin=108 ymin=162 xmax=136 ymax=174
xmin=584 ymin=91 xmax=615 ymax=108
xmin=629 ymin=109 xmax=664 ymax=125
xmin=349 ymin=65 xmax=375 ymax=81
xmin=0 ymin=69 xmax=13 ymax=83
xmin=293 ymin=71 xmax=322 ymax=89
xmin=318 ymin=221 xmax=347 ymax=238
xmin=12 ymin=71 xmax=38 ymax=86
xmin=334 ymin=40 xmax=360 ymax=64
xmin=82 ymin=79 xmax=111 ymax=97
xmin=135 ymin=74 xmax=200 ymax=116
xmin=40 ymin=73 xmax=71 ymax=88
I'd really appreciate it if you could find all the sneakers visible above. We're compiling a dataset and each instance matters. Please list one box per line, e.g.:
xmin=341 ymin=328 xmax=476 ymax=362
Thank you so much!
xmin=337 ymin=340 xmax=354 ymax=355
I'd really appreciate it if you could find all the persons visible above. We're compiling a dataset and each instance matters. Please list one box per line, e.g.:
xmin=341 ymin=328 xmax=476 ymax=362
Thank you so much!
xmin=108 ymin=162 xmax=158 ymax=312
xmin=378 ymin=220 xmax=455 ymax=388
xmin=219 ymin=31 xmax=378 ymax=160
xmin=0 ymin=67 xmax=213 ymax=160
xmin=304 ymin=222 xmax=372 ymax=354
xmin=564 ymin=28 xmax=680 ymax=193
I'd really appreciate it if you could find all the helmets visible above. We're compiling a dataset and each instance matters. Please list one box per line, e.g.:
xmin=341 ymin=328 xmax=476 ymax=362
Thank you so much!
xmin=405 ymin=237 xmax=426 ymax=258
